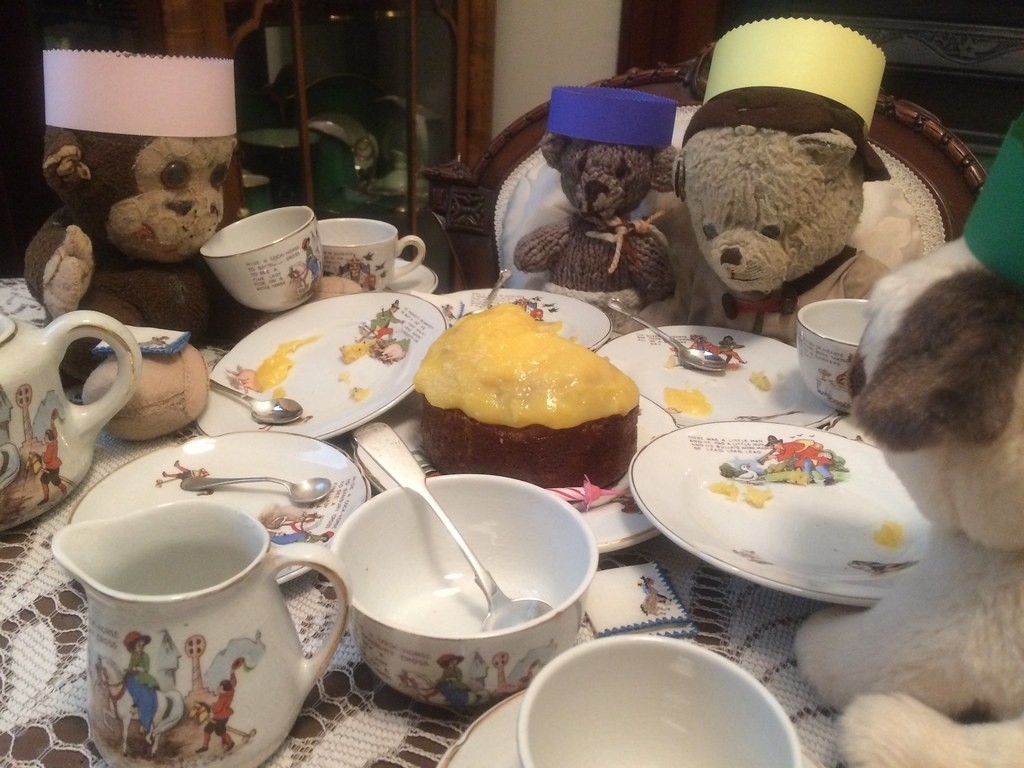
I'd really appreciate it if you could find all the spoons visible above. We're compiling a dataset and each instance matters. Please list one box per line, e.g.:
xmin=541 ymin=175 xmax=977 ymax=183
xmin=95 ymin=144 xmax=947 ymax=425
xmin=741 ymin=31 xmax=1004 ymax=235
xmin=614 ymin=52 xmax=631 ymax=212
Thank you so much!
xmin=352 ymin=420 xmax=554 ymax=635
xmin=181 ymin=475 xmax=333 ymax=503
xmin=205 ymin=379 xmax=303 ymax=424
xmin=607 ymin=297 xmax=729 ymax=372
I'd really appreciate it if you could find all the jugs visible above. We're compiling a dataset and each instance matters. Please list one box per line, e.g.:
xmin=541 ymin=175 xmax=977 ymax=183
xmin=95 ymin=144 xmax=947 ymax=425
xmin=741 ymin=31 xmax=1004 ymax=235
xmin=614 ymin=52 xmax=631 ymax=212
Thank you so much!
xmin=49 ymin=499 xmax=349 ymax=768
xmin=0 ymin=309 xmax=144 ymax=528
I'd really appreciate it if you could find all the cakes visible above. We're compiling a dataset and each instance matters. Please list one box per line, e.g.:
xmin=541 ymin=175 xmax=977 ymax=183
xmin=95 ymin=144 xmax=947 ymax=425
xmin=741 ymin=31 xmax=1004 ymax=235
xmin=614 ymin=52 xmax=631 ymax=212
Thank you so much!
xmin=413 ymin=306 xmax=641 ymax=489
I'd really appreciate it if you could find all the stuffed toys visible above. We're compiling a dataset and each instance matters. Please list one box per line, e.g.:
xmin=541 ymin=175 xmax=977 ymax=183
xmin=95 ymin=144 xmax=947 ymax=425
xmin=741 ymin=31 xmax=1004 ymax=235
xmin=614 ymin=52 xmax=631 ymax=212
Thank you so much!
xmin=798 ymin=237 xmax=1024 ymax=768
xmin=515 ymin=84 xmax=683 ymax=334
xmin=24 ymin=48 xmax=365 ymax=441
xmin=620 ymin=14 xmax=892 ymax=353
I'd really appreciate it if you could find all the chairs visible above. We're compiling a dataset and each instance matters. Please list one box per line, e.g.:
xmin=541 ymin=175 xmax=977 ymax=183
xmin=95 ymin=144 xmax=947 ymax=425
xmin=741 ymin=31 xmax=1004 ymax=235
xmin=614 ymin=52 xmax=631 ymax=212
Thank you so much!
xmin=422 ymin=39 xmax=990 ymax=294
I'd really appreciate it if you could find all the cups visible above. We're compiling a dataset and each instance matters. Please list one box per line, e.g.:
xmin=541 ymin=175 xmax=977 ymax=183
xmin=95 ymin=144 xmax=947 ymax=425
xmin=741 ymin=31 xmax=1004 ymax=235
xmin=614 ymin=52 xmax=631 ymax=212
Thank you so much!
xmin=199 ymin=205 xmax=325 ymax=313
xmin=316 ymin=217 xmax=427 ymax=291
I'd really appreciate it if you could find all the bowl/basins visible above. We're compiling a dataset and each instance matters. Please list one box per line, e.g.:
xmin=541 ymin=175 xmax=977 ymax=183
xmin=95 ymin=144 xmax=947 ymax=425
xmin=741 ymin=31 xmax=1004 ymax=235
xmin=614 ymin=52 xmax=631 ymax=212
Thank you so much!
xmin=515 ymin=633 xmax=803 ymax=768
xmin=796 ymin=298 xmax=871 ymax=413
xmin=239 ymin=128 xmax=320 ymax=179
xmin=328 ymin=472 xmax=600 ymax=706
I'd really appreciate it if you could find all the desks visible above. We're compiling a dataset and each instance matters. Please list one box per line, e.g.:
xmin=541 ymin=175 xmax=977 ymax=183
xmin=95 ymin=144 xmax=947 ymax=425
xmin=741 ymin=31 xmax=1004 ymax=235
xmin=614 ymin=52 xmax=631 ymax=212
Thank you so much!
xmin=0 ymin=334 xmax=879 ymax=767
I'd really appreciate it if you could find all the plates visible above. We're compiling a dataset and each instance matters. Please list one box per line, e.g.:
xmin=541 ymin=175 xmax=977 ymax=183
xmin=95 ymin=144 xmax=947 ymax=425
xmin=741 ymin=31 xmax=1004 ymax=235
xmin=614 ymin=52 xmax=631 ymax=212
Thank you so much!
xmin=67 ymin=286 xmax=935 ymax=768
xmin=435 ymin=686 xmax=522 ymax=768
xmin=393 ymin=257 xmax=438 ymax=293
